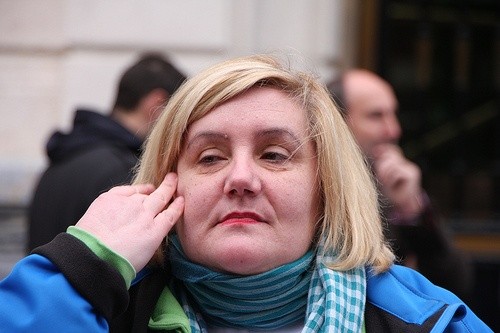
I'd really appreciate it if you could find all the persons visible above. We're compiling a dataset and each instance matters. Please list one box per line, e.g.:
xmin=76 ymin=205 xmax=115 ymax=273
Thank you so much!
xmin=0 ymin=54 xmax=494 ymax=333
xmin=25 ymin=54 xmax=187 ymax=333
xmin=325 ymin=67 xmax=469 ymax=332
